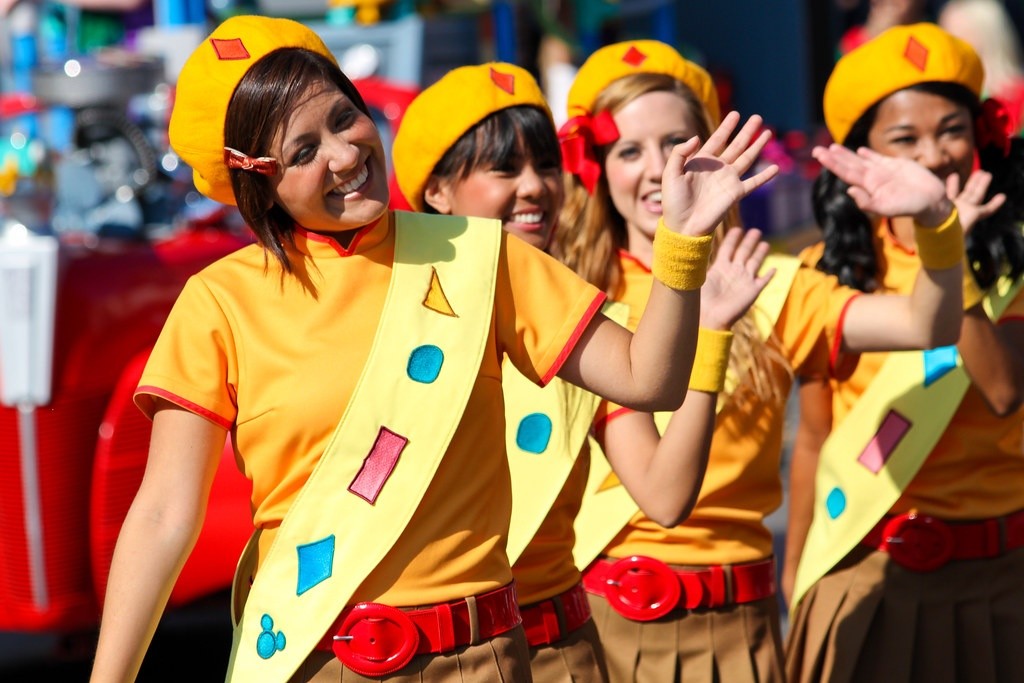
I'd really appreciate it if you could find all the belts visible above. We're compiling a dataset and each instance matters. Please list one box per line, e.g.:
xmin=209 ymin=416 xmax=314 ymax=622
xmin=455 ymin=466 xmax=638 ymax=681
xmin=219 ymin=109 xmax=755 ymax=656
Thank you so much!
xmin=861 ymin=510 xmax=1023 ymax=576
xmin=583 ymin=556 xmax=775 ymax=622
xmin=315 ymin=580 xmax=522 ymax=677
xmin=521 ymin=583 xmax=591 ymax=646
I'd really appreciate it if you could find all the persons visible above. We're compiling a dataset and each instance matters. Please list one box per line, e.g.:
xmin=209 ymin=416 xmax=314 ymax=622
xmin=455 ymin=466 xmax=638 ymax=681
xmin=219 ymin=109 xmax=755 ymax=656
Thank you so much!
xmin=548 ymin=37 xmax=964 ymax=682
xmin=782 ymin=21 xmax=1024 ymax=683
xmin=391 ymin=60 xmax=777 ymax=683
xmin=88 ymin=14 xmax=777 ymax=682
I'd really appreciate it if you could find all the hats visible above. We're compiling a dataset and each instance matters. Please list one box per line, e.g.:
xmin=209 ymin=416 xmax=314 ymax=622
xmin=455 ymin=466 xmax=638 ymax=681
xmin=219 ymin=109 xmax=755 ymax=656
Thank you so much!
xmin=391 ymin=60 xmax=555 ymax=211
xmin=166 ymin=14 xmax=340 ymax=209
xmin=822 ymin=23 xmax=985 ymax=144
xmin=565 ymin=40 xmax=721 ymax=136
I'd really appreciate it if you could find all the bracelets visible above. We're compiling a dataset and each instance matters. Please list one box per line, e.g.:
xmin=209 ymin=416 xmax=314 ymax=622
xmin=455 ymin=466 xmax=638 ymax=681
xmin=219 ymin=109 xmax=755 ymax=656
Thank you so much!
xmin=915 ymin=212 xmax=963 ymax=270
xmin=963 ymin=257 xmax=981 ymax=311
xmin=689 ymin=327 xmax=732 ymax=395
xmin=649 ymin=219 xmax=714 ymax=289
